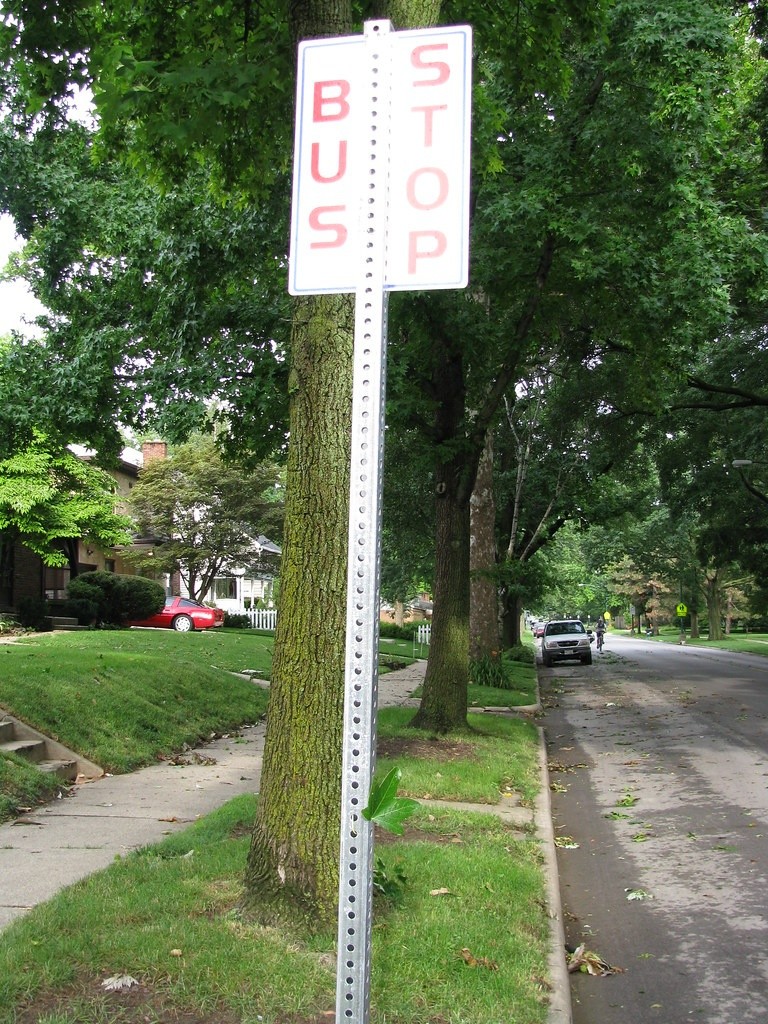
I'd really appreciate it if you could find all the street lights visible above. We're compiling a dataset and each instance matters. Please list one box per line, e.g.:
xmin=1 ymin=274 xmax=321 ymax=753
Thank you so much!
xmin=578 ymin=584 xmax=609 ymax=629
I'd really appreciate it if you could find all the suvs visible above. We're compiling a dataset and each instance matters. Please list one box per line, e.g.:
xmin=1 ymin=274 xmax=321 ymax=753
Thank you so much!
xmin=542 ymin=620 xmax=593 ymax=667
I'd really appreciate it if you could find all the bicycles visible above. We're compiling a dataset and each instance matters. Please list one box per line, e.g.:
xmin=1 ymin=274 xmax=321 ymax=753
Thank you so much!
xmin=596 ymin=630 xmax=606 ymax=652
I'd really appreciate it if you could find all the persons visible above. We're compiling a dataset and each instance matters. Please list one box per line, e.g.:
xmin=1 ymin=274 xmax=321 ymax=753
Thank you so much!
xmin=596 ymin=618 xmax=606 ymax=649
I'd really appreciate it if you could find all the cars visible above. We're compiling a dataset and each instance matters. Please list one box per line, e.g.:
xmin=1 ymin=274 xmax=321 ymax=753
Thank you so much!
xmin=120 ymin=596 xmax=225 ymax=631
xmin=530 ymin=620 xmax=548 ymax=639
xmin=587 ymin=634 xmax=596 ymax=644
